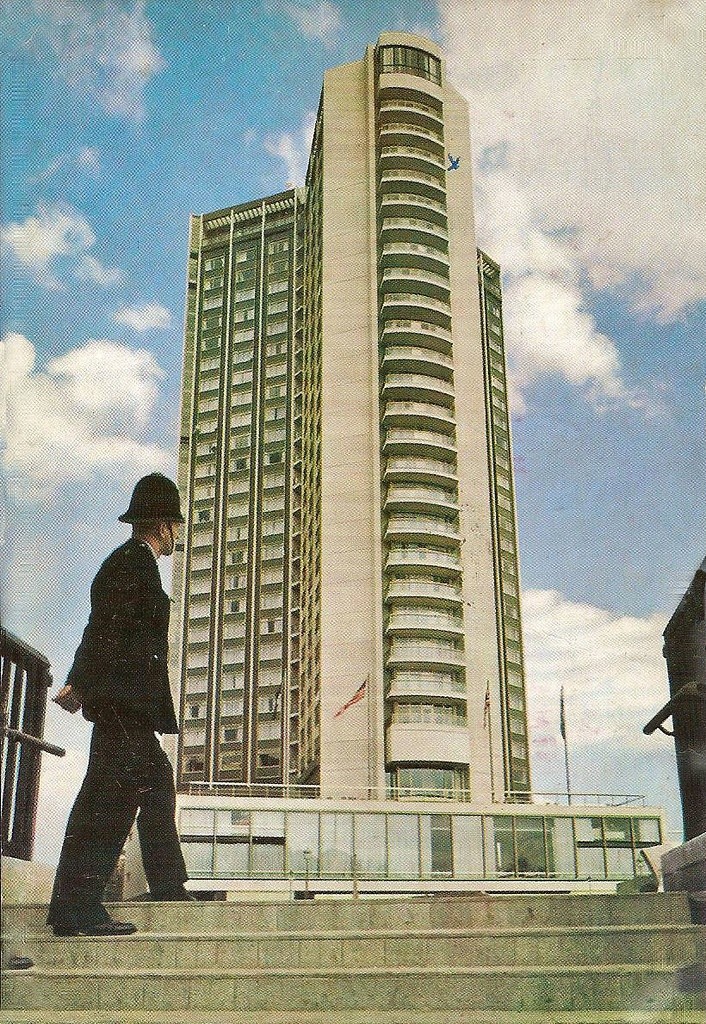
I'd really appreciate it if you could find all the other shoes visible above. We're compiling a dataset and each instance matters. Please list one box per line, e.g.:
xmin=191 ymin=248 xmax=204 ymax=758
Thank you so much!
xmin=6 ymin=955 xmax=32 ymax=969
xmin=53 ymin=921 xmax=136 ymax=936
xmin=150 ymin=887 xmax=195 ymax=901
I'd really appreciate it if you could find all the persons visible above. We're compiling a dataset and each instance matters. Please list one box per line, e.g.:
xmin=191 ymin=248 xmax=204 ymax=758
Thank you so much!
xmin=48 ymin=474 xmax=204 ymax=935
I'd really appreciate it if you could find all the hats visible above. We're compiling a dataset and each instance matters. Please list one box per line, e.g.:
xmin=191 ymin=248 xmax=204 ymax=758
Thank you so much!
xmin=117 ymin=474 xmax=186 ymax=526
